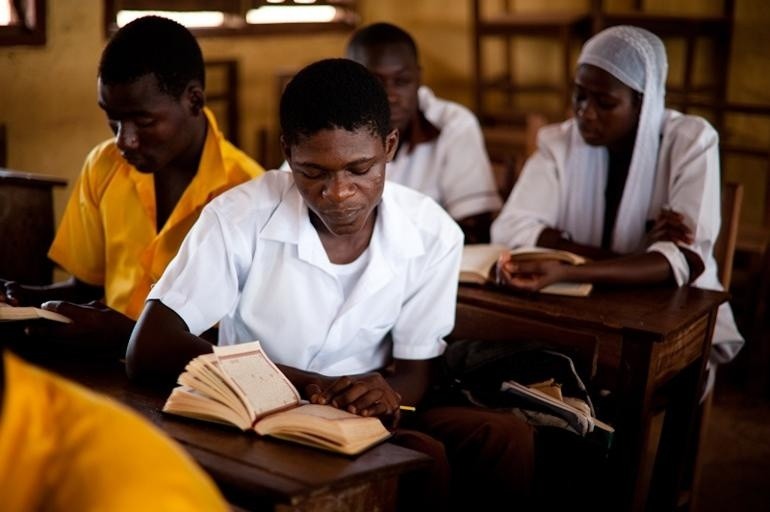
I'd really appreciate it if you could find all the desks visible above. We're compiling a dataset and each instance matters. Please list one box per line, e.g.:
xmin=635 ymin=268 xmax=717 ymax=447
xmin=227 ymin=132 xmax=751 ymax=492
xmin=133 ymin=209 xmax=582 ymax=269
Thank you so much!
xmin=449 ymin=238 xmax=732 ymax=512
xmin=0 ymin=171 xmax=69 ymax=283
xmin=79 ymin=362 xmax=435 ymax=511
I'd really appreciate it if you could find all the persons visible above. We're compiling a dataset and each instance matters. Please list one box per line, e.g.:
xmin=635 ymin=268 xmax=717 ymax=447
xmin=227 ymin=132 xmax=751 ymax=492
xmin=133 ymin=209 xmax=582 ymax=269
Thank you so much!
xmin=488 ymin=23 xmax=744 ymax=512
xmin=122 ymin=55 xmax=544 ymax=511
xmin=0 ymin=349 xmax=232 ymax=511
xmin=1 ymin=13 xmax=267 ymax=376
xmin=279 ymin=19 xmax=503 ymax=247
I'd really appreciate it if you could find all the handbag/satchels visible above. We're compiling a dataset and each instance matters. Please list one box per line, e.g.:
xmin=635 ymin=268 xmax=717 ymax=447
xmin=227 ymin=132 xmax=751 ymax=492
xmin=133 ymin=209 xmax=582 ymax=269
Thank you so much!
xmin=463 ymin=344 xmax=616 ymax=440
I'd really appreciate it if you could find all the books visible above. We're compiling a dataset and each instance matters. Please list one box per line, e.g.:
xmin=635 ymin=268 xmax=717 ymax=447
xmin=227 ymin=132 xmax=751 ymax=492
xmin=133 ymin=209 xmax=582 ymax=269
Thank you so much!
xmin=162 ymin=336 xmax=394 ymax=462
xmin=459 ymin=236 xmax=598 ymax=300
xmin=0 ymin=296 xmax=75 ymax=336
xmin=501 ymin=372 xmax=614 ymax=461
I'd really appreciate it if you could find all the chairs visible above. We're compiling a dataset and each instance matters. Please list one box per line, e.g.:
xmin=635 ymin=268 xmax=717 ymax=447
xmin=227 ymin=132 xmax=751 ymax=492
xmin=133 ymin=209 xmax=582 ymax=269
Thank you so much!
xmin=714 ymin=182 xmax=745 ymax=293
